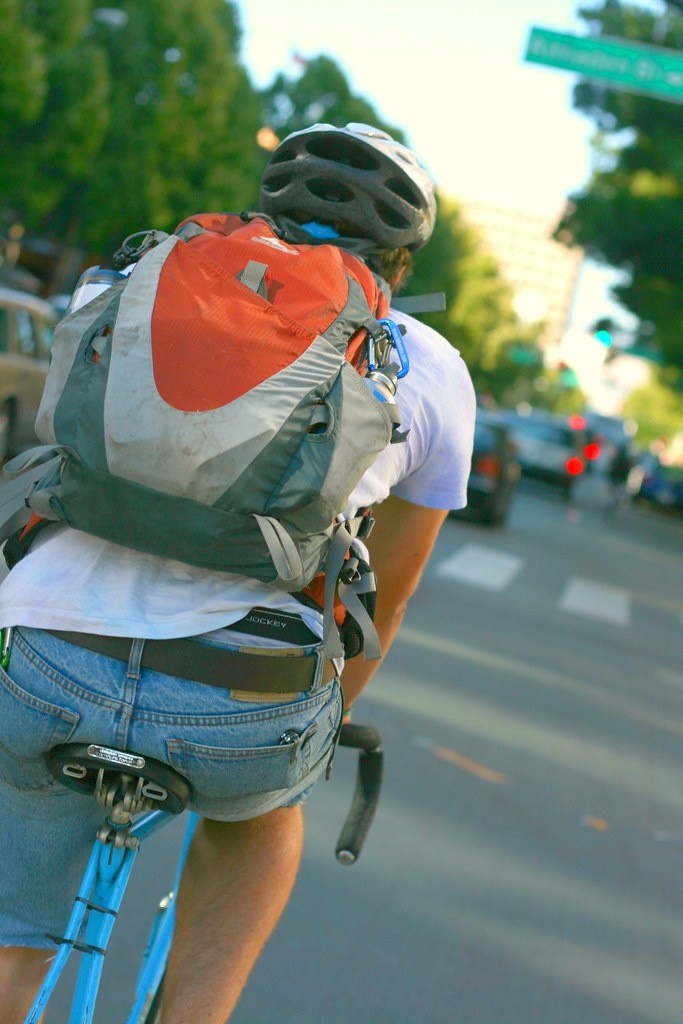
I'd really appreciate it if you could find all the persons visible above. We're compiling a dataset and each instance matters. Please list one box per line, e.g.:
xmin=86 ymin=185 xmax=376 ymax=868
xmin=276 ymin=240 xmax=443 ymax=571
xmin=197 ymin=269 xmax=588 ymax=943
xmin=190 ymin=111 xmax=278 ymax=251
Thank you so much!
xmin=0 ymin=122 xmax=476 ymax=1024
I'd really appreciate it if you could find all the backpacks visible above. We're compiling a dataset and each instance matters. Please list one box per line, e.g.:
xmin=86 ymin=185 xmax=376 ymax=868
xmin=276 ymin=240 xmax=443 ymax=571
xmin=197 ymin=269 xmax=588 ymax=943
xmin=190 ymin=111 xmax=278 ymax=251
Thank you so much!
xmin=27 ymin=211 xmax=409 ymax=592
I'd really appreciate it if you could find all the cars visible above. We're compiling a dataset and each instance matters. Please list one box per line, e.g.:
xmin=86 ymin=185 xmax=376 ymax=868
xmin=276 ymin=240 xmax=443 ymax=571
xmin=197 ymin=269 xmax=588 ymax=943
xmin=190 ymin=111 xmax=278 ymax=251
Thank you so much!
xmin=634 ymin=457 xmax=683 ymax=512
xmin=443 ymin=408 xmax=522 ymax=527
xmin=579 ymin=408 xmax=628 ymax=477
xmin=0 ymin=290 xmax=62 ymax=468
xmin=18 ymin=292 xmax=73 ymax=359
xmin=506 ymin=407 xmax=590 ymax=500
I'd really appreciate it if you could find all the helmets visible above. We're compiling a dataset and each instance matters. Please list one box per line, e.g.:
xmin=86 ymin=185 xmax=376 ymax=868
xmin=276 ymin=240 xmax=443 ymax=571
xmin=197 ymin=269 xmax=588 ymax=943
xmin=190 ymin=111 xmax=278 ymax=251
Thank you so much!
xmin=260 ymin=122 xmax=437 ymax=251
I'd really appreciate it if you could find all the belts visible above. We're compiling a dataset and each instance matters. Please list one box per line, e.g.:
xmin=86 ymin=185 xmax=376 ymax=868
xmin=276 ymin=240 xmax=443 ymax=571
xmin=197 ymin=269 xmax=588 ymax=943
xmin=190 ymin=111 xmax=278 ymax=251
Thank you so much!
xmin=47 ymin=629 xmax=337 ymax=693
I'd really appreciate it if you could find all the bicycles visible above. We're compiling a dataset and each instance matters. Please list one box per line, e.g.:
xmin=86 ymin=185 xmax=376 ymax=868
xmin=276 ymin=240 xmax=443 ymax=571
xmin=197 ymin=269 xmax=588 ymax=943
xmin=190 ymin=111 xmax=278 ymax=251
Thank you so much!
xmin=0 ymin=722 xmax=386 ymax=1023
